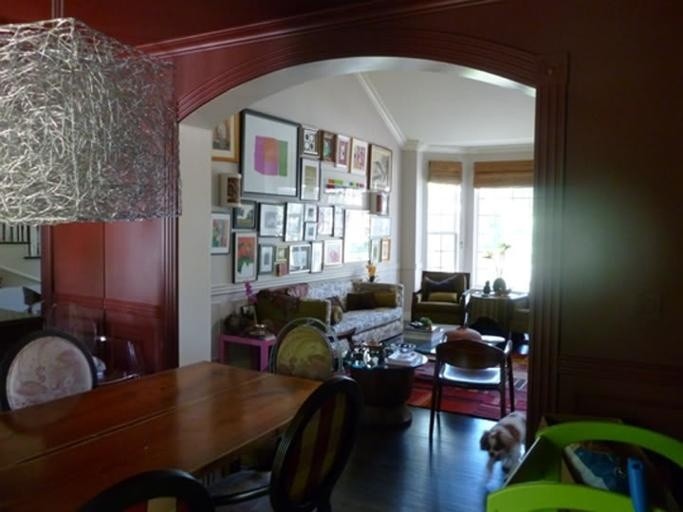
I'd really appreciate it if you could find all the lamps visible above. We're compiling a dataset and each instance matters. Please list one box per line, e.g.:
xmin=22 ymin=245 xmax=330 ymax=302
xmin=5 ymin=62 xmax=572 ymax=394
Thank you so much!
xmin=218 ymin=172 xmax=241 ymax=209
xmin=0 ymin=1 xmax=182 ymax=228
xmin=370 ymin=192 xmax=388 ymax=216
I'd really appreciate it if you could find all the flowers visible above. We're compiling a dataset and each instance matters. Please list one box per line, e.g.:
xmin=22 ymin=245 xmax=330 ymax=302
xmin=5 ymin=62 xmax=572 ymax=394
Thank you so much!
xmin=482 ymin=243 xmax=512 ymax=277
xmin=245 ymin=281 xmax=257 ymax=306
xmin=365 ymin=260 xmax=376 ymax=277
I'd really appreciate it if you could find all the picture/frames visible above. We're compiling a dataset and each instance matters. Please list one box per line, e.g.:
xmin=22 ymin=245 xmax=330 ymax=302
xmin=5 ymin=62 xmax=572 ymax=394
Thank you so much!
xmin=300 ymin=158 xmax=320 ymax=201
xmin=370 ymin=239 xmax=382 ymax=263
xmin=304 ymin=204 xmax=319 ymax=223
xmin=258 ymin=244 xmax=276 ymax=274
xmin=319 ymin=206 xmax=334 ymax=235
xmin=241 ymin=109 xmax=302 ymax=204
xmin=300 ymin=125 xmax=321 ymax=160
xmin=289 ymin=243 xmax=312 ymax=274
xmin=310 ymin=241 xmax=323 ymax=273
xmin=233 ymin=232 xmax=257 ymax=284
xmin=240 ymin=306 xmax=249 ymax=316
xmin=369 ymin=144 xmax=392 ymax=192
xmin=233 ymin=200 xmax=258 ymax=229
xmin=337 ymin=135 xmax=351 ymax=169
xmin=304 ymin=223 xmax=317 ymax=242
xmin=344 ymin=209 xmax=368 ymax=262
xmin=324 ymin=240 xmax=343 ymax=266
xmin=381 ymin=239 xmax=391 ymax=261
xmin=258 ymin=203 xmax=285 ymax=237
xmin=321 ymin=129 xmax=336 ymax=162
xmin=276 ymin=244 xmax=289 ymax=261
xmin=285 ymin=203 xmax=305 ymax=241
xmin=351 ymin=138 xmax=368 ymax=177
xmin=369 ymin=216 xmax=393 ymax=238
xmin=210 ymin=111 xmax=240 ymax=162
xmin=211 ymin=207 xmax=233 ymax=256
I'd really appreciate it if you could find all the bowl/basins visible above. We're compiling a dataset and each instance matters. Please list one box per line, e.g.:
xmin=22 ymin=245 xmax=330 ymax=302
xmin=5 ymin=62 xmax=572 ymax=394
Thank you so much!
xmin=391 ymin=343 xmax=417 ymax=357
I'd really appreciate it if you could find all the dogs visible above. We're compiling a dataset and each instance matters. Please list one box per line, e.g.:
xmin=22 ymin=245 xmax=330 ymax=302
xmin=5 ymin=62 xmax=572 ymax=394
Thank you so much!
xmin=478 ymin=410 xmax=526 ymax=484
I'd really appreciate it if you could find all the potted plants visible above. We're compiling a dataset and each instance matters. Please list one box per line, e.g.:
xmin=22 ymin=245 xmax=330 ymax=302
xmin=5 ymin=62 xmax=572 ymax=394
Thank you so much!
xmin=419 ymin=317 xmax=432 ymax=332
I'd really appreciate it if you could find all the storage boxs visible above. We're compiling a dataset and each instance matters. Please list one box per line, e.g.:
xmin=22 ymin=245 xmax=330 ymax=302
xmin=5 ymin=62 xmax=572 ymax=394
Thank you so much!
xmin=537 ymin=415 xmax=680 ymax=512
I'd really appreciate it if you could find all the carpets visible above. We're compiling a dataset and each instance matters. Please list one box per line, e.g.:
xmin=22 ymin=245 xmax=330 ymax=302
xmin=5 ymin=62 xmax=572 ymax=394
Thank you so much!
xmin=331 ymin=354 xmax=528 ymax=421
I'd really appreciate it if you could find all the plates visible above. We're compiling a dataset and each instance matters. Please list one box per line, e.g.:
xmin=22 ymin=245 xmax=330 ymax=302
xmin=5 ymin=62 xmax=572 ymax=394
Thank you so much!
xmin=388 ymin=350 xmax=417 ymax=362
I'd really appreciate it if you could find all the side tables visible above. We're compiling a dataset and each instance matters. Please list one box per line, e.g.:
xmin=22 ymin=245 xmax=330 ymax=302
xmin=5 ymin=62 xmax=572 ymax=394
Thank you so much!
xmin=218 ymin=332 xmax=277 ymax=371
xmin=471 ymin=292 xmax=509 ymax=327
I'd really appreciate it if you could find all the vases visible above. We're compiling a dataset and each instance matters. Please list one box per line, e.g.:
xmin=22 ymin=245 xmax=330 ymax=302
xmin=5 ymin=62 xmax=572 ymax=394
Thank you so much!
xmin=247 ymin=307 xmax=257 ymax=331
xmin=493 ymin=278 xmax=507 ymax=292
xmin=483 ymin=281 xmax=490 ymax=294
xmin=369 ymin=276 xmax=374 ymax=283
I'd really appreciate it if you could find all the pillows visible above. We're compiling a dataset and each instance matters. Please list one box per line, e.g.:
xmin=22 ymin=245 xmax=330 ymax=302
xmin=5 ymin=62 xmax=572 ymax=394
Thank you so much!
xmin=374 ymin=292 xmax=396 ymax=308
xmin=424 ymin=275 xmax=458 ymax=302
xmin=331 ymin=305 xmax=343 ymax=325
xmin=347 ymin=291 xmax=375 ymax=311
xmin=428 ymin=292 xmax=457 ymax=302
xmin=445 ymin=327 xmax=482 ymax=343
xmin=327 ymin=297 xmax=345 ymax=312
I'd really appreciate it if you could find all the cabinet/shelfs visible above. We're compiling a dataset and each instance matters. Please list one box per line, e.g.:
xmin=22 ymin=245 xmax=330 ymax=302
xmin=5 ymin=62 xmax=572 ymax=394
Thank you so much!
xmin=40 ymin=219 xmax=179 ymax=375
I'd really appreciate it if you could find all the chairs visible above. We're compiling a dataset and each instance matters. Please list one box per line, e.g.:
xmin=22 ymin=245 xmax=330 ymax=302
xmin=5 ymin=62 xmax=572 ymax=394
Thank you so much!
xmin=1 ymin=329 xmax=98 ymax=413
xmin=509 ymin=295 xmax=530 ymax=332
xmin=429 ymin=340 xmax=514 ymax=445
xmin=326 ymin=327 xmax=363 ymax=371
xmin=206 ymin=375 xmax=362 ymax=512
xmin=76 ymin=469 xmax=216 ymax=512
xmin=411 ymin=271 xmax=470 ymax=326
xmin=220 ymin=317 xmax=343 ymax=478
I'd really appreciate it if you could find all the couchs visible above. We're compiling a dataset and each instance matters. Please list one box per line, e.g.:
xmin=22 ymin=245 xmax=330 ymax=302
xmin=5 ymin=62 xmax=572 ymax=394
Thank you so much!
xmin=255 ymin=276 xmax=405 ymax=360
